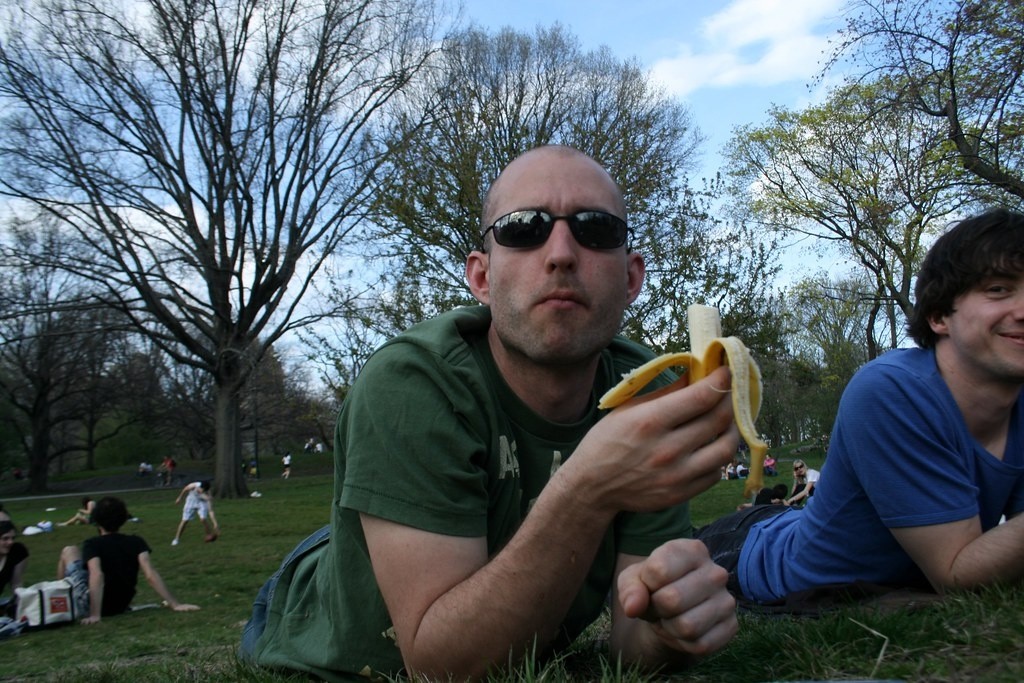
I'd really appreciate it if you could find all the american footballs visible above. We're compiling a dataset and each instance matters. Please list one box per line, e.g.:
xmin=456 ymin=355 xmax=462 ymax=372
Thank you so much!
xmin=204 ymin=533 xmax=217 ymax=544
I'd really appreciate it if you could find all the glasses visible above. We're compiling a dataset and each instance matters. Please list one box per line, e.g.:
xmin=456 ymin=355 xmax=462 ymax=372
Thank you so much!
xmin=480 ymin=210 xmax=636 ymax=254
xmin=794 ymin=464 xmax=804 ymax=471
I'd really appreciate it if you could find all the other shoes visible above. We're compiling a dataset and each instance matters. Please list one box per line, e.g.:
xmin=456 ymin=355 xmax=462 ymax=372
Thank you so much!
xmin=171 ymin=539 xmax=180 ymax=546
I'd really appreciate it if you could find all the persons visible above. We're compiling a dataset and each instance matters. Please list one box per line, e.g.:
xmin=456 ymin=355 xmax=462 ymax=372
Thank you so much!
xmin=138 ymin=454 xmax=185 ymax=488
xmin=240 ymin=145 xmax=740 ymax=682
xmin=281 ymin=451 xmax=292 ymax=479
xmin=719 ymin=449 xmax=821 ymax=514
xmin=55 ymin=495 xmax=203 ymax=626
xmin=56 ymin=496 xmax=96 ymax=527
xmin=170 ymin=480 xmax=222 ymax=546
xmin=241 ymin=457 xmax=260 ymax=480
xmin=304 ymin=434 xmax=323 ymax=455
xmin=684 ymin=206 xmax=1024 ymax=611
xmin=0 ymin=503 xmax=31 ymax=619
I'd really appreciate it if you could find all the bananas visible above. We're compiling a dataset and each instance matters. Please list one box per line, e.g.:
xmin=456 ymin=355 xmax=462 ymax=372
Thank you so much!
xmin=596 ymin=303 xmax=767 ymax=497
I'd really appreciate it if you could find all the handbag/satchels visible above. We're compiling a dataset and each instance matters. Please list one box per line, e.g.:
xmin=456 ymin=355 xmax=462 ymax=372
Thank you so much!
xmin=8 ymin=577 xmax=78 ymax=632
